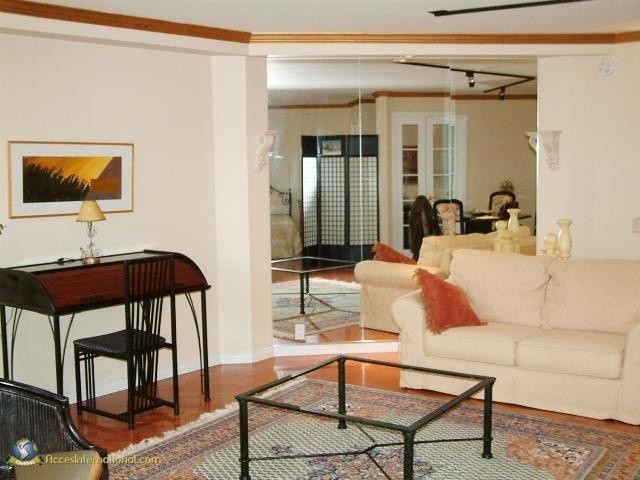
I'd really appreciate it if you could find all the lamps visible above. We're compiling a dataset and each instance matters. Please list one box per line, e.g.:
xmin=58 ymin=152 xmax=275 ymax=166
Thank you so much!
xmin=525 ymin=130 xmax=537 ymax=151
xmin=498 ymin=88 xmax=509 ymax=99
xmin=539 ymin=130 xmax=562 ymax=162
xmin=465 ymin=72 xmax=476 ymax=88
xmin=76 ymin=188 xmax=107 ymax=265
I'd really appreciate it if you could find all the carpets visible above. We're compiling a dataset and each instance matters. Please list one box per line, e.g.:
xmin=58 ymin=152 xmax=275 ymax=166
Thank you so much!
xmin=106 ymin=375 xmax=640 ymax=480
xmin=271 ymin=276 xmax=362 ymax=342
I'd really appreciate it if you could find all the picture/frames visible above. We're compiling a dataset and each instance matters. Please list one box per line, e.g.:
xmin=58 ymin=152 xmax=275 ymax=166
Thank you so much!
xmin=7 ymin=139 xmax=136 ymax=221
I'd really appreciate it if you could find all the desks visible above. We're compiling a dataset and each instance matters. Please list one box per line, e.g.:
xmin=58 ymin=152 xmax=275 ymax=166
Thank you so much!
xmin=1 ymin=250 xmax=212 ymax=402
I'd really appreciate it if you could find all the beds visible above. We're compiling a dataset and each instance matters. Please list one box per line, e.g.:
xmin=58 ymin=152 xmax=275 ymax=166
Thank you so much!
xmin=270 ymin=185 xmax=304 ymax=259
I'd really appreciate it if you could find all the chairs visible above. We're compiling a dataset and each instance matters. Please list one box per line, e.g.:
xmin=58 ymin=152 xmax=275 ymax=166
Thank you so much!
xmin=73 ymin=253 xmax=179 ymax=429
xmin=408 ymin=188 xmax=533 ymax=259
xmin=0 ymin=380 xmax=110 ymax=480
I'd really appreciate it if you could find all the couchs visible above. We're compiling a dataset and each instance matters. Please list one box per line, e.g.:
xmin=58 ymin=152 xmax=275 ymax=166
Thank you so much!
xmin=354 ymin=226 xmax=536 ymax=335
xmin=390 ymin=244 xmax=640 ymax=426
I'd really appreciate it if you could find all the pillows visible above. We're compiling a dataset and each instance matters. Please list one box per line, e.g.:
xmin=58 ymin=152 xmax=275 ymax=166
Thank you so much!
xmin=545 ymin=259 xmax=640 ymax=333
xmin=449 ymin=249 xmax=553 ymax=327
xmin=416 ymin=267 xmax=487 ymax=333
xmin=370 ymin=241 xmax=418 ymax=265
xmin=419 ymin=232 xmax=494 ymax=268
